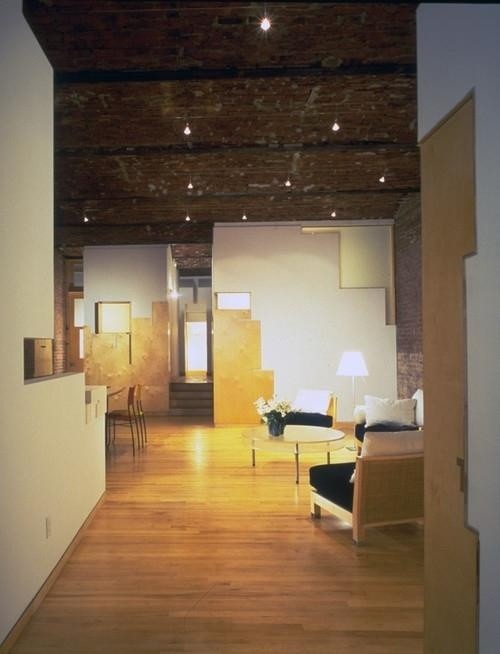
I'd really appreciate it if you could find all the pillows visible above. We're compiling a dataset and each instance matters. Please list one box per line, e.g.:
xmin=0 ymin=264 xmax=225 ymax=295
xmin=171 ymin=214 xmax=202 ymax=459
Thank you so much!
xmin=364 ymin=393 xmax=416 ymax=426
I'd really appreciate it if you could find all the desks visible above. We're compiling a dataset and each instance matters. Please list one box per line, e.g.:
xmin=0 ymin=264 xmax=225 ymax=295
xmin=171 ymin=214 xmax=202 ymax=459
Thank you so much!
xmin=106 ymin=384 xmax=126 ymax=446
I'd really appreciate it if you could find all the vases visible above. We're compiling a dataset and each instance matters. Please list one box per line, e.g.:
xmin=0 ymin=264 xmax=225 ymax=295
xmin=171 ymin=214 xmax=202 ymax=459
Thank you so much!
xmin=268 ymin=413 xmax=284 ymax=436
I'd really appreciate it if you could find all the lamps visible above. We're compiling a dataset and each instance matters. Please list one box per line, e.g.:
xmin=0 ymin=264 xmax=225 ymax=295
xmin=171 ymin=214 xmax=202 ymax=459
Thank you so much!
xmin=336 ymin=351 xmax=370 ymax=451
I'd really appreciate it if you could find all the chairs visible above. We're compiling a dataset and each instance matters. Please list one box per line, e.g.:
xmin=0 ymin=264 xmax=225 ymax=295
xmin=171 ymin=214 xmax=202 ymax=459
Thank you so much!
xmin=286 ymin=389 xmax=336 ymax=428
xmin=109 ymin=384 xmax=140 ymax=456
xmin=113 ymin=384 xmax=149 ymax=446
xmin=354 ymin=389 xmax=425 ymax=461
xmin=309 ymin=429 xmax=429 ymax=546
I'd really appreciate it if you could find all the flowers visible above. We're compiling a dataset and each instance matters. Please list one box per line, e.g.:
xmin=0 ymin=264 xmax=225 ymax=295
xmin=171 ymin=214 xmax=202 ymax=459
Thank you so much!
xmin=253 ymin=393 xmax=297 ymax=423
